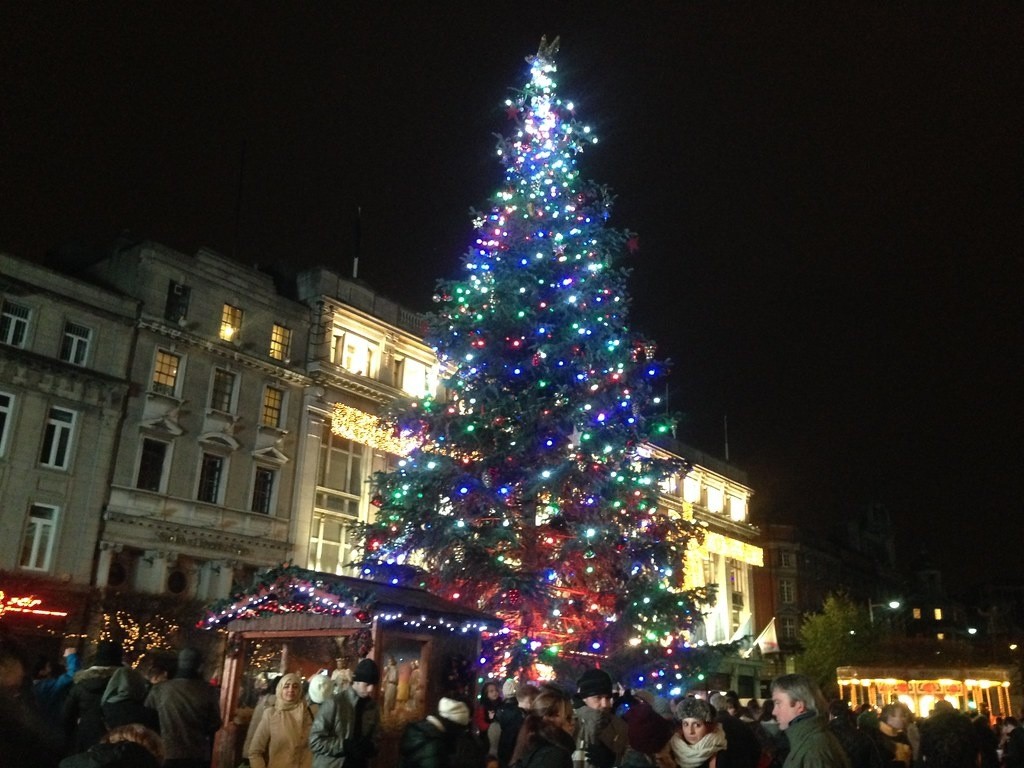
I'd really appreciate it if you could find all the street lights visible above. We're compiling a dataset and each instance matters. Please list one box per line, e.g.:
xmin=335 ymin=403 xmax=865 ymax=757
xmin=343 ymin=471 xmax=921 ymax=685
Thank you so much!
xmin=952 ymin=625 xmax=978 ymax=652
xmin=868 ymin=598 xmax=900 ymax=626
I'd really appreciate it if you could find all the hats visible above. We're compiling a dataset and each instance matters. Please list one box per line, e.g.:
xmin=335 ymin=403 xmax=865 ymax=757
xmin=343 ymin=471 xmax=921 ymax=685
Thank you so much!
xmin=308 ymin=674 xmax=332 ymax=704
xmin=351 ymin=659 xmax=380 ymax=686
xmin=502 ymin=678 xmax=520 ymax=698
xmin=178 ymin=647 xmax=200 ymax=670
xmin=677 ymin=699 xmax=718 ymax=725
xmin=577 ymin=669 xmax=613 ymax=699
xmin=439 ymin=697 xmax=469 ymax=724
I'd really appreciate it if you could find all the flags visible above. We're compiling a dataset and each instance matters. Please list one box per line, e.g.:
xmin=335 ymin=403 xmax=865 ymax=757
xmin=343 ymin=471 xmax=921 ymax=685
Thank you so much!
xmin=758 ymin=621 xmax=780 ymax=655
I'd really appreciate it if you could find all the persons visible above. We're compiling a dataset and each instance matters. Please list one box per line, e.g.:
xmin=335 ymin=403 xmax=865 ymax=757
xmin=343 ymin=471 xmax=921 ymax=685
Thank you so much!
xmin=0 ymin=628 xmax=1024 ymax=768
